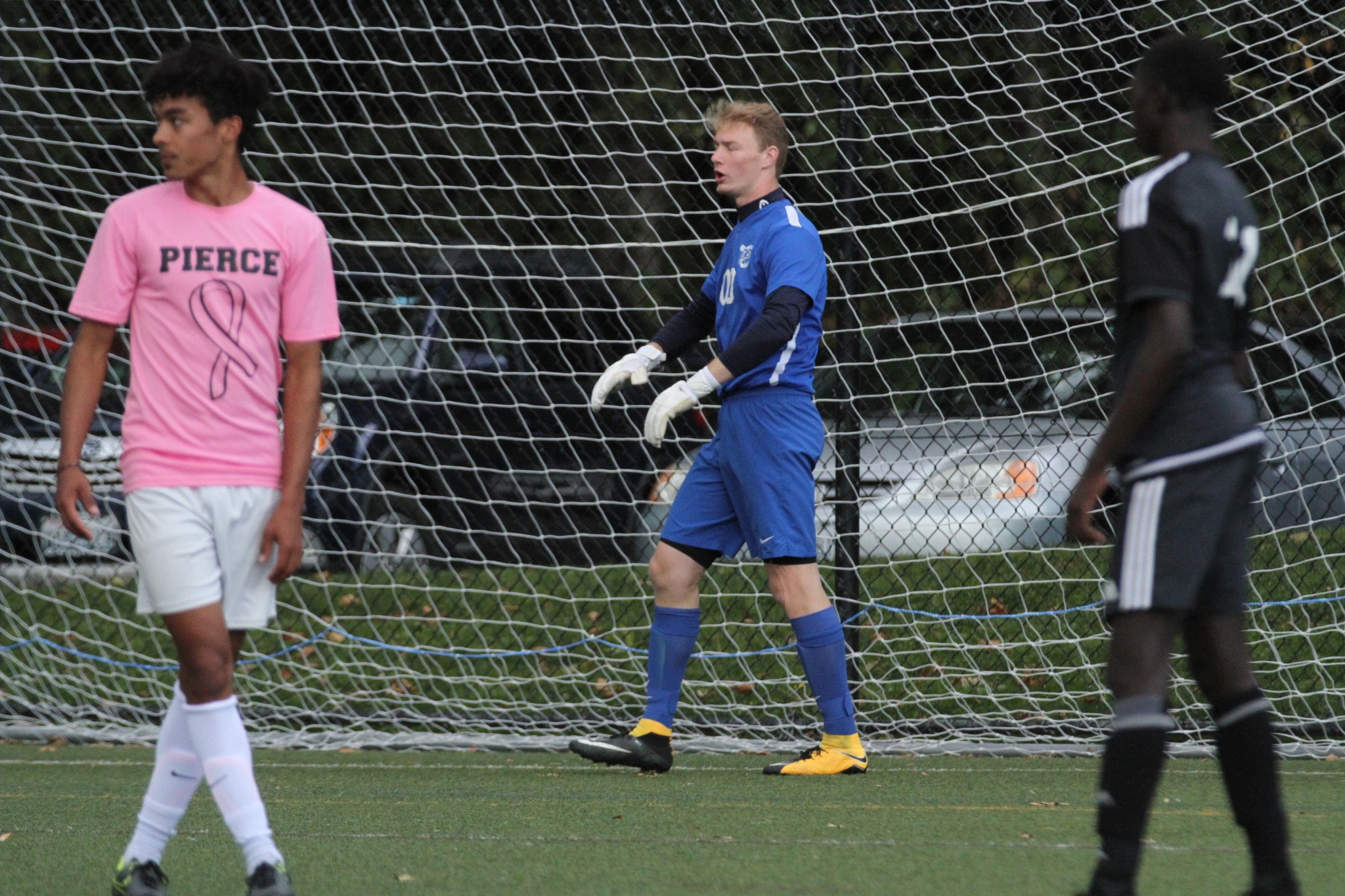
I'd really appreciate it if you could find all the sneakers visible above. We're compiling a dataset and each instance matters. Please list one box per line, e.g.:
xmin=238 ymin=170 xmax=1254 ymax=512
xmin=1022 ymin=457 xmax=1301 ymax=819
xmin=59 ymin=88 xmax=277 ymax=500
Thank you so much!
xmin=246 ymin=862 xmax=292 ymax=896
xmin=762 ymin=746 xmax=869 ymax=776
xmin=111 ymin=857 xmax=169 ymax=896
xmin=569 ymin=731 xmax=674 ymax=775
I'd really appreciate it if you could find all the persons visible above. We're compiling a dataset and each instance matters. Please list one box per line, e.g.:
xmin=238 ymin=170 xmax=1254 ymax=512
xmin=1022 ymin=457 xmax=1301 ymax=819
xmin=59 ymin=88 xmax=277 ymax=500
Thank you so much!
xmin=569 ymin=97 xmax=868 ymax=779
xmin=1060 ymin=32 xmax=1302 ymax=896
xmin=52 ymin=43 xmax=339 ymax=896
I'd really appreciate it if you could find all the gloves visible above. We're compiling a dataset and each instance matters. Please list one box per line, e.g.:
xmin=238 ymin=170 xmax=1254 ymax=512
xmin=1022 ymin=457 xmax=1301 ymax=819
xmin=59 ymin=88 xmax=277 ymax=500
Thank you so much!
xmin=645 ymin=368 xmax=721 ymax=446
xmin=592 ymin=344 xmax=666 ymax=413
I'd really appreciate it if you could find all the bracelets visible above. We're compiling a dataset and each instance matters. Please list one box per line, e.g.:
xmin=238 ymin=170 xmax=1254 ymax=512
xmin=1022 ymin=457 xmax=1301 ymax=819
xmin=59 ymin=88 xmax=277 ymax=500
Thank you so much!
xmin=55 ymin=463 xmax=79 ymax=476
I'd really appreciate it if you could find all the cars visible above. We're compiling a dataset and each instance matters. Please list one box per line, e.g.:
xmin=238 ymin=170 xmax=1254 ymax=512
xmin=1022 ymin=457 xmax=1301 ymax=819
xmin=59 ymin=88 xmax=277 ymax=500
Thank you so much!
xmin=0 ymin=325 xmax=133 ymax=563
xmin=633 ymin=305 xmax=1345 ymax=563
xmin=287 ymin=265 xmax=719 ymax=572
